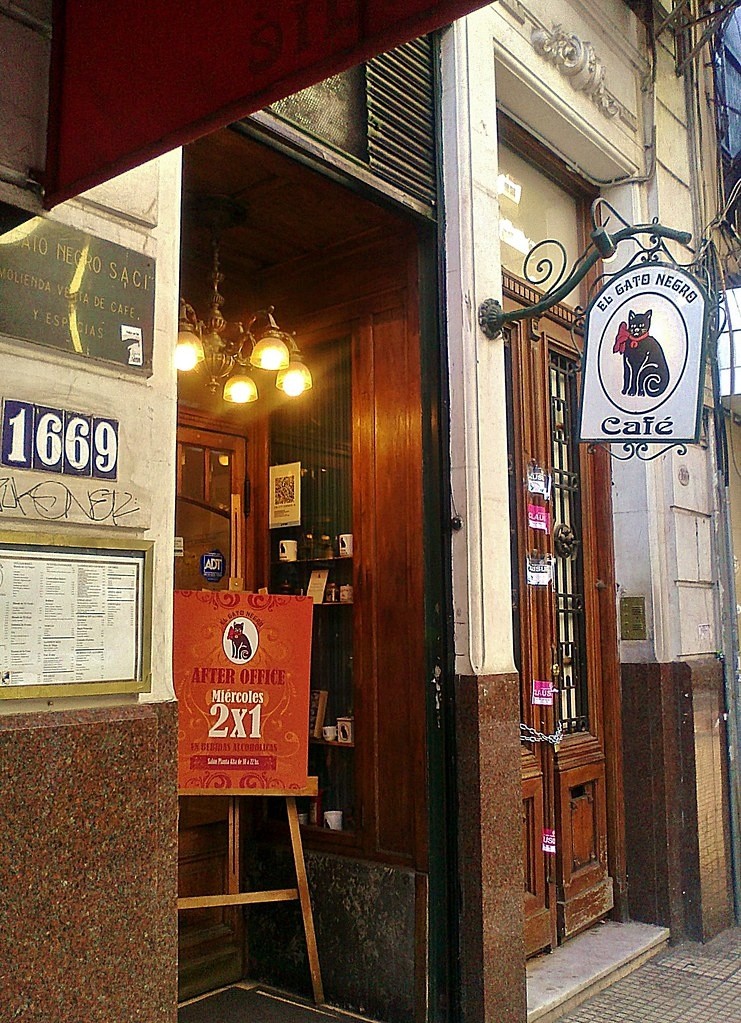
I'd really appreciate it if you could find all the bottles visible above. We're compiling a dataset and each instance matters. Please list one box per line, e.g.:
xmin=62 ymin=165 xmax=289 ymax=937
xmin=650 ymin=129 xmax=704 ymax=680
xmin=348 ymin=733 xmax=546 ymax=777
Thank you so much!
xmin=325 ymin=583 xmax=341 ymax=603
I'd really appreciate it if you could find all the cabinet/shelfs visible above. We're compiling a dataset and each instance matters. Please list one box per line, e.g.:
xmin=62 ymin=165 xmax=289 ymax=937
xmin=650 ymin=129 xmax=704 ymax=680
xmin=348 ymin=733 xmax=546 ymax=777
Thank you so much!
xmin=273 ymin=558 xmax=363 ymax=856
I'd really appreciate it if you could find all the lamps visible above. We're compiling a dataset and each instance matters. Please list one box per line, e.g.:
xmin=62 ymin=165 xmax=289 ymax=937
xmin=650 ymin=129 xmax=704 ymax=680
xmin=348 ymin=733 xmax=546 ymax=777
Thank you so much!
xmin=175 ymin=238 xmax=313 ymax=405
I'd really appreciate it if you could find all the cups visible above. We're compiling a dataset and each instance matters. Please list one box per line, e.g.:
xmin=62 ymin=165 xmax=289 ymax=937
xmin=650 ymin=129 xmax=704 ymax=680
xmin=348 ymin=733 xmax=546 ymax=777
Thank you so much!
xmin=338 ymin=585 xmax=355 ymax=603
xmin=279 ymin=540 xmax=298 ymax=562
xmin=322 ymin=726 xmax=339 ymax=741
xmin=338 ymin=533 xmax=354 ymax=557
xmin=297 ymin=812 xmax=308 ymax=826
xmin=324 ymin=810 xmax=343 ymax=832
xmin=337 ymin=715 xmax=355 ymax=743
